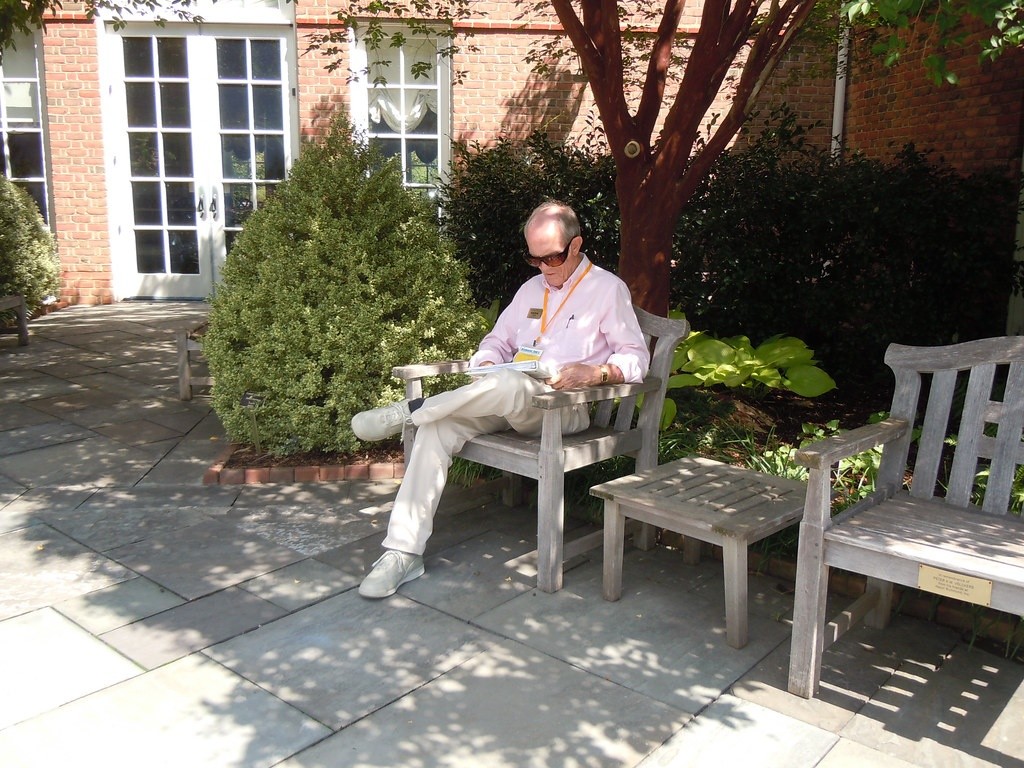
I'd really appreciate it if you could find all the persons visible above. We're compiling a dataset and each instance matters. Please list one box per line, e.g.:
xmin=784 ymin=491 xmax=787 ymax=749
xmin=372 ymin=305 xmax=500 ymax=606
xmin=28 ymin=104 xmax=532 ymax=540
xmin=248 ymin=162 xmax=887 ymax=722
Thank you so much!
xmin=351 ymin=201 xmax=652 ymax=599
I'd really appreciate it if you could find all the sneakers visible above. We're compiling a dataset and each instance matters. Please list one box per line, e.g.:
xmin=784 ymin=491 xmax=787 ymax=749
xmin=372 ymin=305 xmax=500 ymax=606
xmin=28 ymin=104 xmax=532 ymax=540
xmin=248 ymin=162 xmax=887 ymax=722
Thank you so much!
xmin=359 ymin=549 xmax=425 ymax=598
xmin=350 ymin=397 xmax=420 ymax=442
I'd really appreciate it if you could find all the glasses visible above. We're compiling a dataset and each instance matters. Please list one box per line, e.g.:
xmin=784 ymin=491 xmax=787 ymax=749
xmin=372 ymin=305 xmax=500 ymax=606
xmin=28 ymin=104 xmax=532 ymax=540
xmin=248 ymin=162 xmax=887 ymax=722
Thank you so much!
xmin=523 ymin=236 xmax=579 ymax=267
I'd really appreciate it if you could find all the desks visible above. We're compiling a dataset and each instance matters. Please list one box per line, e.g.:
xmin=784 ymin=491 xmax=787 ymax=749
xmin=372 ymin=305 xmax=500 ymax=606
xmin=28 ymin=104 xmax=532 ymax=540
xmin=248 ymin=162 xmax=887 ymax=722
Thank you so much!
xmin=591 ymin=457 xmax=839 ymax=648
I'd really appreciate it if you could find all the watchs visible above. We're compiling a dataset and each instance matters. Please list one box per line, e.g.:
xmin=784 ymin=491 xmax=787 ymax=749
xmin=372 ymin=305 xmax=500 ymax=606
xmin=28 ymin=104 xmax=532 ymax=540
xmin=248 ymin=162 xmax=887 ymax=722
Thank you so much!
xmin=599 ymin=365 xmax=609 ymax=386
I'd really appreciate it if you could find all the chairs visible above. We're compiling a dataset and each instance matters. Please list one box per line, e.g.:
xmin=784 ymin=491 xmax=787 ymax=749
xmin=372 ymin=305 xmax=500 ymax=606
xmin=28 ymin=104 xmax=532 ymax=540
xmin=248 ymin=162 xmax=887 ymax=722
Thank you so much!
xmin=394 ymin=304 xmax=690 ymax=593
xmin=789 ymin=333 xmax=1024 ymax=699
